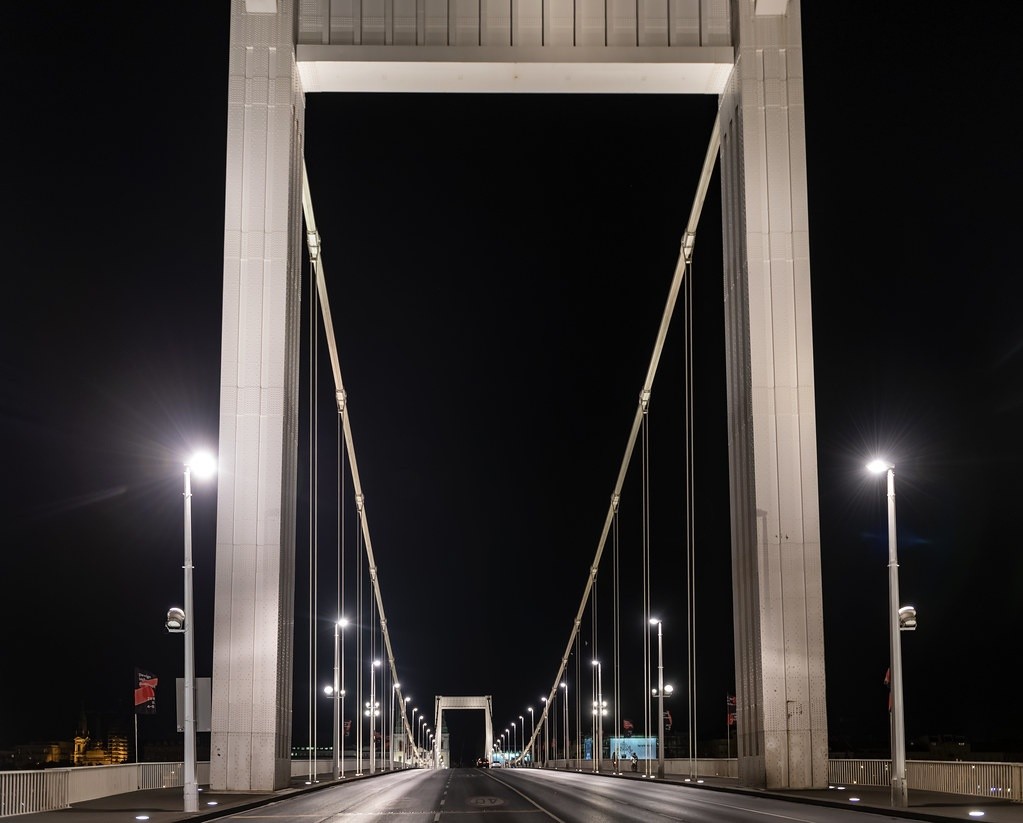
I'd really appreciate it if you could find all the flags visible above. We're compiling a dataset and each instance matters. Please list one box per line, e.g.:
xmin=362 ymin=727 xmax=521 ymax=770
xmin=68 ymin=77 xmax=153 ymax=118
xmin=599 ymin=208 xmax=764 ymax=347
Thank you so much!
xmin=373 ymin=730 xmax=381 ymax=744
xmin=344 ymin=720 xmax=353 ymax=737
xmin=727 ymin=697 xmax=736 ymax=725
xmin=883 ymin=665 xmax=891 ymax=713
xmin=663 ymin=711 xmax=672 ymax=731
xmin=134 ymin=670 xmax=159 ymax=715
xmin=624 ymin=720 xmax=633 ymax=738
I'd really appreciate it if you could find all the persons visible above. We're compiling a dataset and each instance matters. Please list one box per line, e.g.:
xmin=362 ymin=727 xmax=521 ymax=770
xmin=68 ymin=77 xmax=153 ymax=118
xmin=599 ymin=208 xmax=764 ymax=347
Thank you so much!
xmin=612 ymin=752 xmax=618 ymax=775
xmin=631 ymin=752 xmax=638 ymax=772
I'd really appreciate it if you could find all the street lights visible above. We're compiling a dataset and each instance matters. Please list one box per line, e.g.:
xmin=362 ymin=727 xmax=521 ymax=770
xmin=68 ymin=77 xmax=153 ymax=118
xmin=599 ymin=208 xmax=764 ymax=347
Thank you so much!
xmin=492 ymin=722 xmax=517 ymax=768
xmin=520 ymin=716 xmax=524 ymax=767
xmin=865 ymin=459 xmax=917 ymax=791
xmin=541 ymin=697 xmax=549 ymax=768
xmin=528 ymin=708 xmax=534 ymax=767
xmin=559 ymin=682 xmax=570 ymax=768
xmin=401 ymin=697 xmax=435 ymax=769
xmin=649 ymin=618 xmax=673 ymax=778
xmin=365 ymin=660 xmax=381 ymax=774
xmin=389 ymin=683 xmax=400 ymax=770
xmin=323 ymin=620 xmax=348 ymax=780
xmin=163 ymin=452 xmax=218 ymax=811
xmin=591 ymin=659 xmax=607 ymax=773
